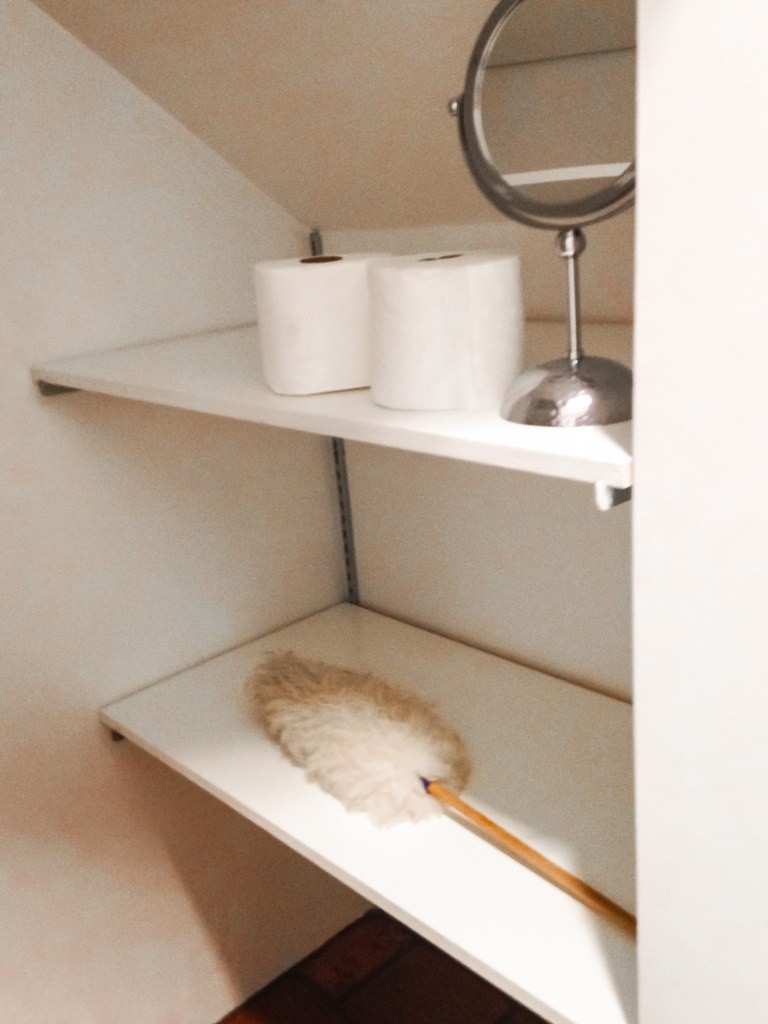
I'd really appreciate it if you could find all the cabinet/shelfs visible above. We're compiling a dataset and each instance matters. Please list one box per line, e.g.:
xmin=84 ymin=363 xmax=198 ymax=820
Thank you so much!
xmin=36 ymin=225 xmax=639 ymax=1024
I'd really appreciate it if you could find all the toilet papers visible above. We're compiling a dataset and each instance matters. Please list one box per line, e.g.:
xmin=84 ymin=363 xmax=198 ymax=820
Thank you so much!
xmin=252 ymin=253 xmax=372 ymax=391
xmin=368 ymin=250 xmax=524 ymax=412
